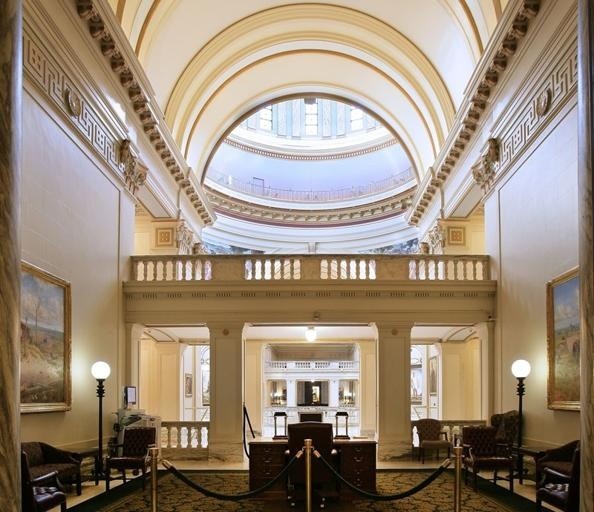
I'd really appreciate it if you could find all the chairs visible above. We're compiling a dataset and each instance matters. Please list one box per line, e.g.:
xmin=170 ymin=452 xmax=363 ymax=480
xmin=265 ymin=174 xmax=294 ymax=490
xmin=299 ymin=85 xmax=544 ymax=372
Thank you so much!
xmin=284 ymin=423 xmax=342 ymax=512
xmin=534 ymin=446 xmax=579 ymax=512
xmin=415 ymin=418 xmax=452 ymax=465
xmin=104 ymin=427 xmax=156 ymax=497
xmin=21 ymin=451 xmax=67 ymax=511
xmin=461 ymin=426 xmax=513 ymax=493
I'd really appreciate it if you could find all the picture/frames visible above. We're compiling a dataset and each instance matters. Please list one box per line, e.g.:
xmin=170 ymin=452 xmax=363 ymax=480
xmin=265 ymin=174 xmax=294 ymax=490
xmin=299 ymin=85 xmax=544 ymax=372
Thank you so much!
xmin=545 ymin=266 xmax=580 ymax=411
xmin=156 ymin=228 xmax=173 ymax=247
xmin=448 ymin=227 xmax=465 ymax=245
xmin=19 ymin=260 xmax=72 ymax=412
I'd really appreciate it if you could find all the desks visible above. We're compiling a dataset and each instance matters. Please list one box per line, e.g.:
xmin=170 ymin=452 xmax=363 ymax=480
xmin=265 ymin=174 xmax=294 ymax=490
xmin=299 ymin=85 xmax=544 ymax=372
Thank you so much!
xmin=515 ymin=441 xmax=566 ymax=499
xmin=56 ymin=442 xmax=99 ymax=495
xmin=248 ymin=437 xmax=377 ymax=502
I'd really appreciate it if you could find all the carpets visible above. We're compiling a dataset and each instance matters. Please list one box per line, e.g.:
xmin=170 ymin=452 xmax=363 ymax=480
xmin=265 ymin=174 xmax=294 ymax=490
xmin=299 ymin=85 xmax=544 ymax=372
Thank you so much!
xmin=66 ymin=470 xmax=558 ymax=512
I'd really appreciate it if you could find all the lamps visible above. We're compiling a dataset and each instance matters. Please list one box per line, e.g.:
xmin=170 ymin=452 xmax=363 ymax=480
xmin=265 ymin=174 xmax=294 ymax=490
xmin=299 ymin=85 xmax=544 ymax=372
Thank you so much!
xmin=90 ymin=359 xmax=110 ymax=481
xmin=510 ymin=358 xmax=531 ymax=484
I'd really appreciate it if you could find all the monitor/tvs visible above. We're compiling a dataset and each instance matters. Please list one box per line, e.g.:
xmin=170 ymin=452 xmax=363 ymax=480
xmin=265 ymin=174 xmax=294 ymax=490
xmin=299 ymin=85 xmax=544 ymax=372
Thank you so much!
xmin=124 ymin=387 xmax=136 ymax=407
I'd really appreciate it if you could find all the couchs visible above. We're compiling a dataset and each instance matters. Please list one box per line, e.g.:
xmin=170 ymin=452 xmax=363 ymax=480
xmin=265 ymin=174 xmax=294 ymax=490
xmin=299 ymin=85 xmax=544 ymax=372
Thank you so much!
xmin=534 ymin=439 xmax=579 ymax=489
xmin=22 ymin=442 xmax=80 ymax=498
